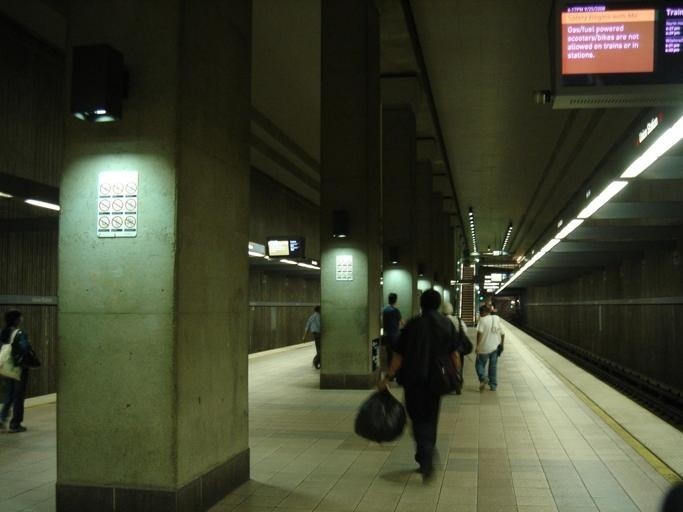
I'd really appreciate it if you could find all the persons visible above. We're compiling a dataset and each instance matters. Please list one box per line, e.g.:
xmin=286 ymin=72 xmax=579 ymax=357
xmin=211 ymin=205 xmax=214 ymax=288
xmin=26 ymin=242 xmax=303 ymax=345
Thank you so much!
xmin=443 ymin=301 xmax=474 ymax=393
xmin=376 ymin=289 xmax=463 ymax=482
xmin=0 ymin=305 xmax=43 ymax=434
xmin=381 ymin=292 xmax=404 ymax=366
xmin=301 ymin=305 xmax=322 ymax=370
xmin=474 ymin=303 xmax=505 ymax=392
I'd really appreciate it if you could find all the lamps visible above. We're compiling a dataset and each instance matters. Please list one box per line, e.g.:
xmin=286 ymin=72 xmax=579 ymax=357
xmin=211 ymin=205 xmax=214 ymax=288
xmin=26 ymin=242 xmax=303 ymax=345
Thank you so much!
xmin=1 ymin=189 xmax=60 ymax=211
xmin=248 ymin=240 xmax=319 ymax=270
xmin=495 ymin=104 xmax=683 ymax=294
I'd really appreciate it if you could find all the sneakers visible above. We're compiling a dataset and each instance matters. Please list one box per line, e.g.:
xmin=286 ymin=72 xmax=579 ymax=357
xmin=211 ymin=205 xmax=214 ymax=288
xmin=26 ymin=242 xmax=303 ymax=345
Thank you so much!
xmin=415 ymin=453 xmax=434 ymax=474
xmin=477 ymin=381 xmax=497 ymax=392
xmin=8 ymin=425 xmax=27 ymax=433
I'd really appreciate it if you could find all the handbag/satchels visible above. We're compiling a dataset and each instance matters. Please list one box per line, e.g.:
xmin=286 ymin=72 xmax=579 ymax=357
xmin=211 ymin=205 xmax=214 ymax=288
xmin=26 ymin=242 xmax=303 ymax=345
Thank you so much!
xmin=428 ymin=356 xmax=462 ymax=394
xmin=0 ymin=342 xmax=23 ymax=382
xmin=456 ymin=336 xmax=472 ymax=355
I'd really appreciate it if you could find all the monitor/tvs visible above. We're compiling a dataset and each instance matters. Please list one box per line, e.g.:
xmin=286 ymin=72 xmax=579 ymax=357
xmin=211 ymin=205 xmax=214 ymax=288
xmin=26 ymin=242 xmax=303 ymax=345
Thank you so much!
xmin=549 ymin=0 xmax=683 ymax=109
xmin=266 ymin=236 xmax=305 ymax=260
